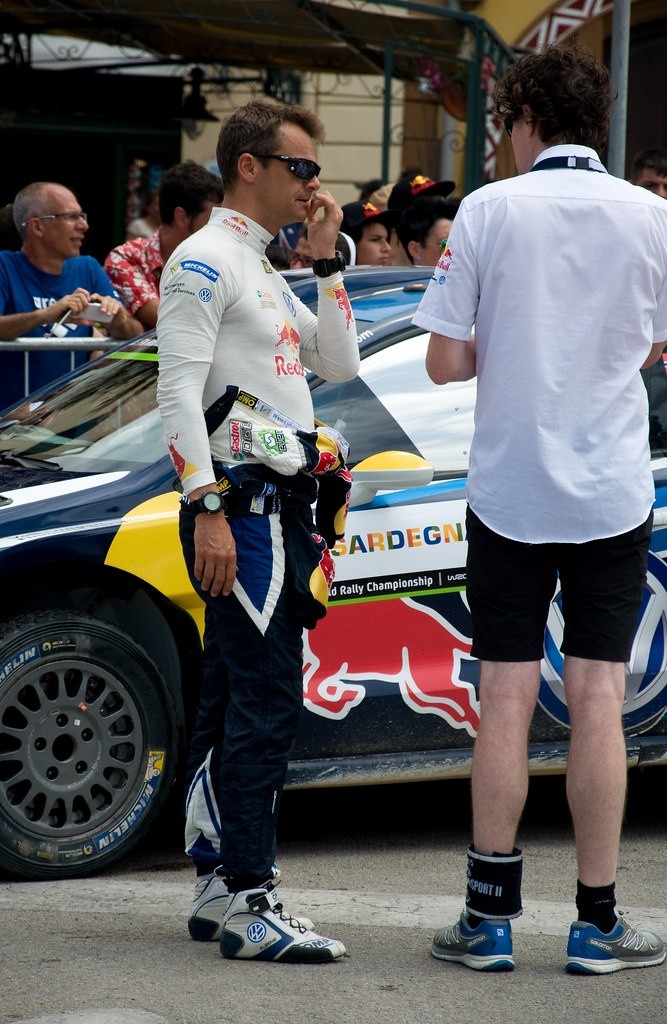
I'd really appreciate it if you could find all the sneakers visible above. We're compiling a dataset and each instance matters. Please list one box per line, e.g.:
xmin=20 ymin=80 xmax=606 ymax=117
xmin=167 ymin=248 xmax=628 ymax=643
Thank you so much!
xmin=431 ymin=912 xmax=516 ymax=971
xmin=188 ymin=876 xmax=315 ymax=940
xmin=220 ymin=888 xmax=346 ymax=963
xmin=564 ymin=912 xmax=666 ymax=975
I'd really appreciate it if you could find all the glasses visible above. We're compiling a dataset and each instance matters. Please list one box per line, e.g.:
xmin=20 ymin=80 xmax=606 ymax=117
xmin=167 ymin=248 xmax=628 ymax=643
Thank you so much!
xmin=256 ymin=153 xmax=322 ymax=182
xmin=21 ymin=212 xmax=87 ymax=229
xmin=505 ymin=112 xmax=523 ymax=136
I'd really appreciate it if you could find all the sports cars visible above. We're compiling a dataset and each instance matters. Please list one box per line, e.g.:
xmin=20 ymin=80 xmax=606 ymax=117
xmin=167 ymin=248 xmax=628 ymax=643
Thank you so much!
xmin=0 ymin=263 xmax=667 ymax=879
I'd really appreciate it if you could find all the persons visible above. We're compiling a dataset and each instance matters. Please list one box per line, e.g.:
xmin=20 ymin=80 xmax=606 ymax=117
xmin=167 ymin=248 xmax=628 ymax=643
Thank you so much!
xmin=157 ymin=102 xmax=360 ymax=964
xmin=0 ymin=183 xmax=143 ymax=413
xmin=628 ymin=146 xmax=667 ymax=200
xmin=103 ymin=160 xmax=223 ymax=329
xmin=410 ymin=42 xmax=667 ymax=974
xmin=265 ymin=168 xmax=456 ymax=272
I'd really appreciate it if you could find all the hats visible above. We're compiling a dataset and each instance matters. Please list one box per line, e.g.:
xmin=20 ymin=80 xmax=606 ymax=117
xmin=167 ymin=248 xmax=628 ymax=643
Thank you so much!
xmin=340 ymin=202 xmax=396 ymax=237
xmin=387 ymin=172 xmax=456 ymax=211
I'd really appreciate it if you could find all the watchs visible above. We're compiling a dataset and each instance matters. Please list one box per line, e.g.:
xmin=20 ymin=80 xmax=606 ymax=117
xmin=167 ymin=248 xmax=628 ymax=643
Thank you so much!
xmin=185 ymin=491 xmax=224 ymax=515
xmin=312 ymin=251 xmax=346 ymax=278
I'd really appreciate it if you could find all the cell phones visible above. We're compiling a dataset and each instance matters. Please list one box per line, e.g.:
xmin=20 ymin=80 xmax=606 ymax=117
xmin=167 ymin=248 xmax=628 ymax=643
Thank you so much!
xmin=46 ymin=302 xmax=114 ymax=338
